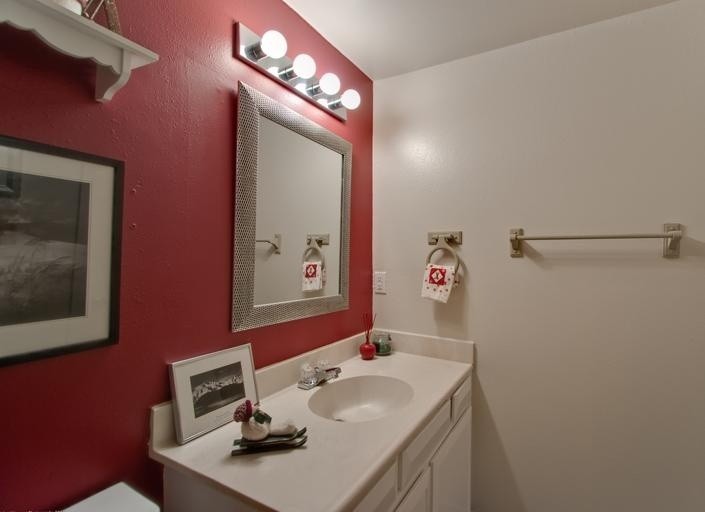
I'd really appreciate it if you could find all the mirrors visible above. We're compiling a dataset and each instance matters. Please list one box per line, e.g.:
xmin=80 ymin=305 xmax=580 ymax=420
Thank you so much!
xmin=232 ymin=80 xmax=349 ymax=333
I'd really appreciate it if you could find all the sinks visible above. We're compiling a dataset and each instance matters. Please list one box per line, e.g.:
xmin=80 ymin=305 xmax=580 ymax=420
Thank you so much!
xmin=306 ymin=374 xmax=415 ymax=421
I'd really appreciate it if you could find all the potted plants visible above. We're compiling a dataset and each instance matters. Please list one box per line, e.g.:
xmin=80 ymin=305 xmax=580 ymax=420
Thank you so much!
xmin=359 ymin=311 xmax=378 ymax=361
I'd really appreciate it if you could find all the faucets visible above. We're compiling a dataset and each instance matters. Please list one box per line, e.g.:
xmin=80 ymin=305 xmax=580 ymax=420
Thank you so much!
xmin=314 ymin=366 xmax=342 ymax=384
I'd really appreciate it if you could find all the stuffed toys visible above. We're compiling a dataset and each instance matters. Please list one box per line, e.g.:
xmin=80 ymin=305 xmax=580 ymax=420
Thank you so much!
xmin=231 ymin=399 xmax=311 ymax=458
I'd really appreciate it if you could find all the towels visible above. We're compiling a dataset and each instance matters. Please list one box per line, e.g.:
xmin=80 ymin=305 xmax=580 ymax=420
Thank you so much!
xmin=421 ymin=263 xmax=458 ymax=304
xmin=301 ymin=260 xmax=323 ymax=293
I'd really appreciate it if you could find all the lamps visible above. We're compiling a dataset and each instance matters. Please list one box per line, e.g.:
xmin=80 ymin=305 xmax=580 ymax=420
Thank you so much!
xmin=233 ymin=22 xmax=362 ymax=121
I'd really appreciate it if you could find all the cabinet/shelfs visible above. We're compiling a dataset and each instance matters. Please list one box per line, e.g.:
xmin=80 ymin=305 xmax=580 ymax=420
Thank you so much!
xmin=353 ymin=372 xmax=472 ymax=512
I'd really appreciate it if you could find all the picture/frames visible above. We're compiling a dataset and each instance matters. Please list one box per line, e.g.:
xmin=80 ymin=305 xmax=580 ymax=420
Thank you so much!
xmin=168 ymin=341 xmax=260 ymax=445
xmin=0 ymin=135 xmax=125 ymax=367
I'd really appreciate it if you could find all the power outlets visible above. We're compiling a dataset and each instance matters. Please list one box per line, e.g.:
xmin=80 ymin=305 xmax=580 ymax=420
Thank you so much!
xmin=373 ymin=272 xmax=387 ymax=294
